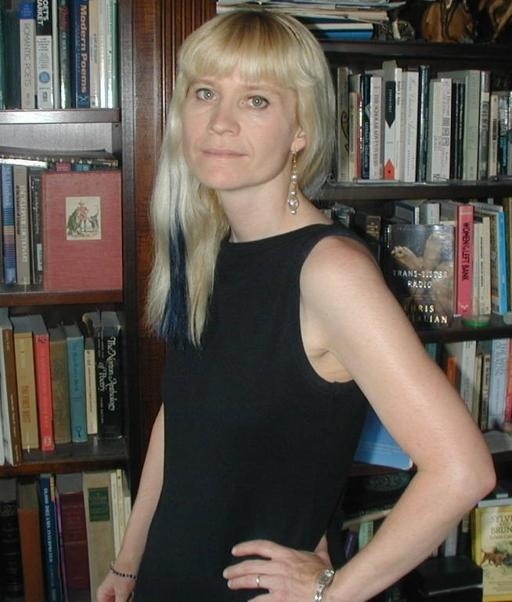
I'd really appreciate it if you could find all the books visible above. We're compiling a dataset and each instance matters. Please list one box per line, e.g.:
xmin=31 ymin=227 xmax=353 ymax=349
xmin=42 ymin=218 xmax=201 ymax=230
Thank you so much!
xmin=217 ymin=1 xmax=512 ymax=328
xmin=2 ymin=1 xmax=123 ymax=294
xmin=0 ymin=306 xmax=132 ymax=602
xmin=325 ymin=337 xmax=511 ymax=602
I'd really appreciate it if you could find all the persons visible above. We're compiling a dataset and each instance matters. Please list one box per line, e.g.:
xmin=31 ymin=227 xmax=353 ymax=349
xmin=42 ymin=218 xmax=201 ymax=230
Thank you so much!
xmin=95 ymin=9 xmax=496 ymax=602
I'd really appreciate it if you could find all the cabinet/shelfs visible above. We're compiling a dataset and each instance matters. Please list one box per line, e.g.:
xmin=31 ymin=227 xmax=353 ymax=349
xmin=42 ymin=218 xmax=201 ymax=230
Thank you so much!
xmin=0 ymin=1 xmax=512 ymax=602
